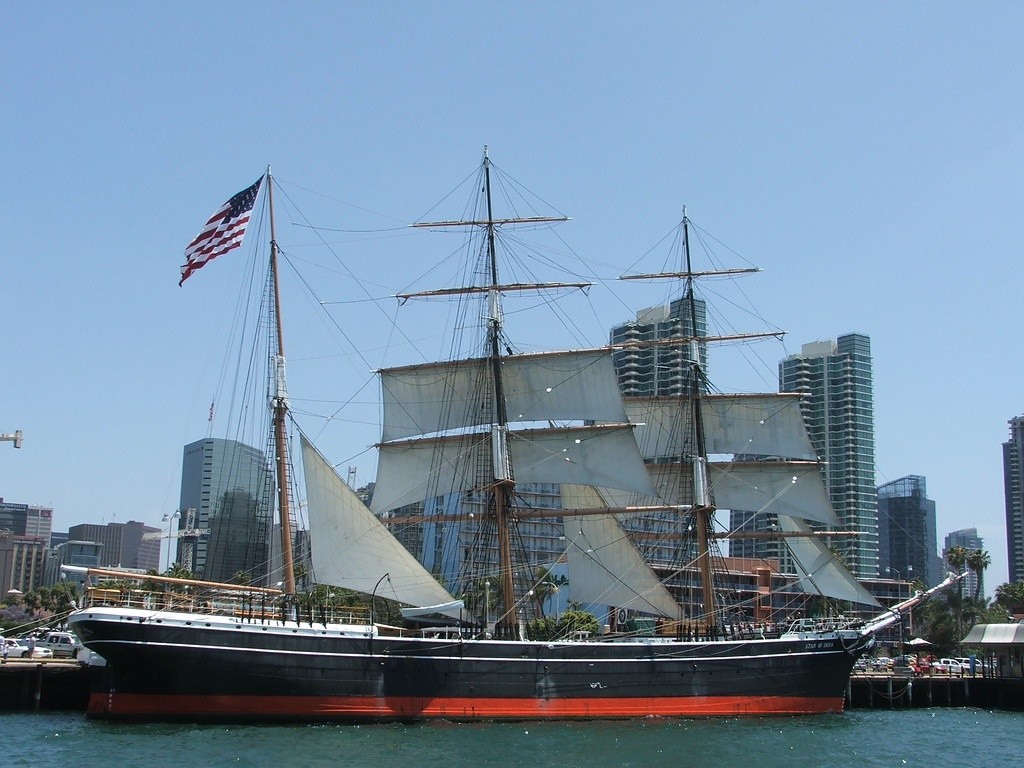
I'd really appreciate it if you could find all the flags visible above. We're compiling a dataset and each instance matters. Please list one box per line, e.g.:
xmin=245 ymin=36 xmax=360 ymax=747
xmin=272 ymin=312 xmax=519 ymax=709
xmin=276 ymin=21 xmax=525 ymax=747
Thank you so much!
xmin=179 ymin=172 xmax=265 ymax=288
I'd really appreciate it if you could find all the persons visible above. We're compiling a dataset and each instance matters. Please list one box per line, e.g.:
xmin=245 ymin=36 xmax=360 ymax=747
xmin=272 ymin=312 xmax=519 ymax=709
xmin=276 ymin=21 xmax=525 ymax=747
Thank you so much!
xmin=4 ymin=634 xmax=36 ymax=659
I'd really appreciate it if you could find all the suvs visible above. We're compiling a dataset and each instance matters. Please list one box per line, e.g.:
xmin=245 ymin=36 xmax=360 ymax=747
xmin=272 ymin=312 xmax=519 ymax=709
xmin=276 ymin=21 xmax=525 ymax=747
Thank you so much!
xmin=956 ymin=658 xmax=993 ymax=676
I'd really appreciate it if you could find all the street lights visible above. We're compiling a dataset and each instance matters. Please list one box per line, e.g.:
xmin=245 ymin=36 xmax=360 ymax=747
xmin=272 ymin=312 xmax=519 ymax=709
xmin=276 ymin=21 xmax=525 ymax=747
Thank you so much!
xmin=713 ymin=589 xmax=742 ymax=624
xmin=161 ymin=509 xmax=182 ymax=592
xmin=542 ymin=582 xmax=559 ymax=624
xmin=884 ymin=565 xmax=913 ymax=643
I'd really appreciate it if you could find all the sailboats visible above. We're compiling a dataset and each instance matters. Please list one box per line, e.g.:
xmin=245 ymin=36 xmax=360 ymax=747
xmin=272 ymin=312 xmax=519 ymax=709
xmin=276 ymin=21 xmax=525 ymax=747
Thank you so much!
xmin=67 ymin=145 xmax=971 ymax=719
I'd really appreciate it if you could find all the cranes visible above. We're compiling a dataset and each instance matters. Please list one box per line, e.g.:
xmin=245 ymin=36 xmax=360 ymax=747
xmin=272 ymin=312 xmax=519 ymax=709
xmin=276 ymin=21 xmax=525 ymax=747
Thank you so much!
xmin=141 ymin=507 xmax=212 ymax=596
xmin=296 ymin=467 xmax=365 ymax=510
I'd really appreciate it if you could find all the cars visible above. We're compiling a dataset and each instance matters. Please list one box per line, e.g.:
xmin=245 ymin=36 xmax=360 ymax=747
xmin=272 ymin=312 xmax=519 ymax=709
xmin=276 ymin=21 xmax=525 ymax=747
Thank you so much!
xmin=853 ymin=662 xmax=866 ymax=671
xmin=860 ymin=655 xmax=928 ymax=671
xmin=4 ymin=638 xmax=52 ymax=657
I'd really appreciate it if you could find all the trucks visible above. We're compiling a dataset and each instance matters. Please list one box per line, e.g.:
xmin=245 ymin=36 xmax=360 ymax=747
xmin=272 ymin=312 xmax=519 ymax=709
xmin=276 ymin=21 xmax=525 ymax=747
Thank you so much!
xmin=929 ymin=658 xmax=962 ymax=673
xmin=25 ymin=632 xmax=82 ymax=658
